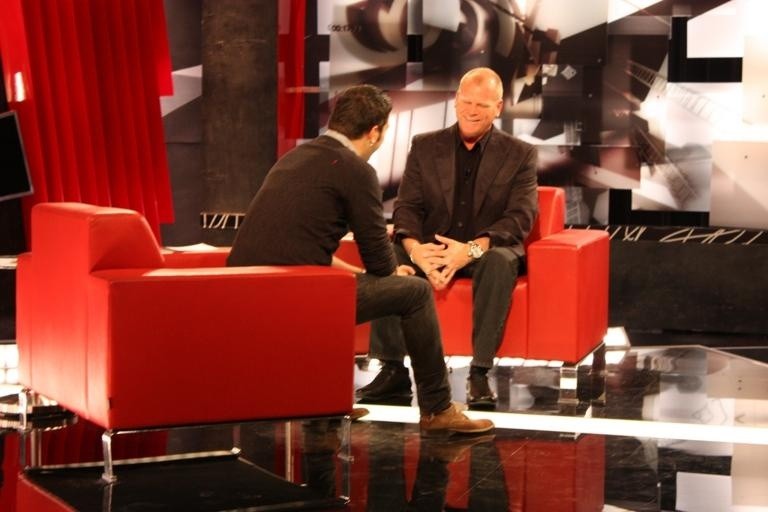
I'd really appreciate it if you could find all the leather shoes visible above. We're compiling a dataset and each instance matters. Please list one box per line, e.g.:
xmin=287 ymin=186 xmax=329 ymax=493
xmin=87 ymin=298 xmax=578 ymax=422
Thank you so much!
xmin=419 ymin=402 xmax=496 ymax=439
xmin=355 ymin=365 xmax=413 ymax=400
xmin=465 ymin=375 xmax=498 ymax=406
xmin=352 ymin=407 xmax=369 ymax=420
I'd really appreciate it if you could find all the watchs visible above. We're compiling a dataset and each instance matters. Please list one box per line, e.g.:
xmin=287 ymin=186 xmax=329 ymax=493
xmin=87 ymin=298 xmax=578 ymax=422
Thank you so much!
xmin=467 ymin=241 xmax=484 ymax=259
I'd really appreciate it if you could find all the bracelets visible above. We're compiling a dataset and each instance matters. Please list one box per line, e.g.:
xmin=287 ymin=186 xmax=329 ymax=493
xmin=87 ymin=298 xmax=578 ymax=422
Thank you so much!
xmin=409 ymin=244 xmax=416 ymax=264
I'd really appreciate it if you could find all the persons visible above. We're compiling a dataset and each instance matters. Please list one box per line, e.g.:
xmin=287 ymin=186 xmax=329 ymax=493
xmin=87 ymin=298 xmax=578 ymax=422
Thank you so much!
xmin=224 ymin=84 xmax=494 ymax=441
xmin=354 ymin=67 xmax=538 ymax=408
xmin=368 ymin=419 xmax=510 ymax=512
xmin=303 ymin=425 xmax=496 ymax=512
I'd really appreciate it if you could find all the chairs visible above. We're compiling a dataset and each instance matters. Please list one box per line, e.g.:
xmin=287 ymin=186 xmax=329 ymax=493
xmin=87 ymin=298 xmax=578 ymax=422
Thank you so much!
xmin=13 ymin=426 xmax=358 ymax=512
xmin=11 ymin=200 xmax=362 ymax=505
xmin=269 ymin=418 xmax=605 ymax=512
xmin=329 ymin=179 xmax=614 ymax=405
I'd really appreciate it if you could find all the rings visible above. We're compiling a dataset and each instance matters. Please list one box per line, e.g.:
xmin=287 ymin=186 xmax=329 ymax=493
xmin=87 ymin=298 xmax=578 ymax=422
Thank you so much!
xmin=425 ymin=272 xmax=429 ymax=277
